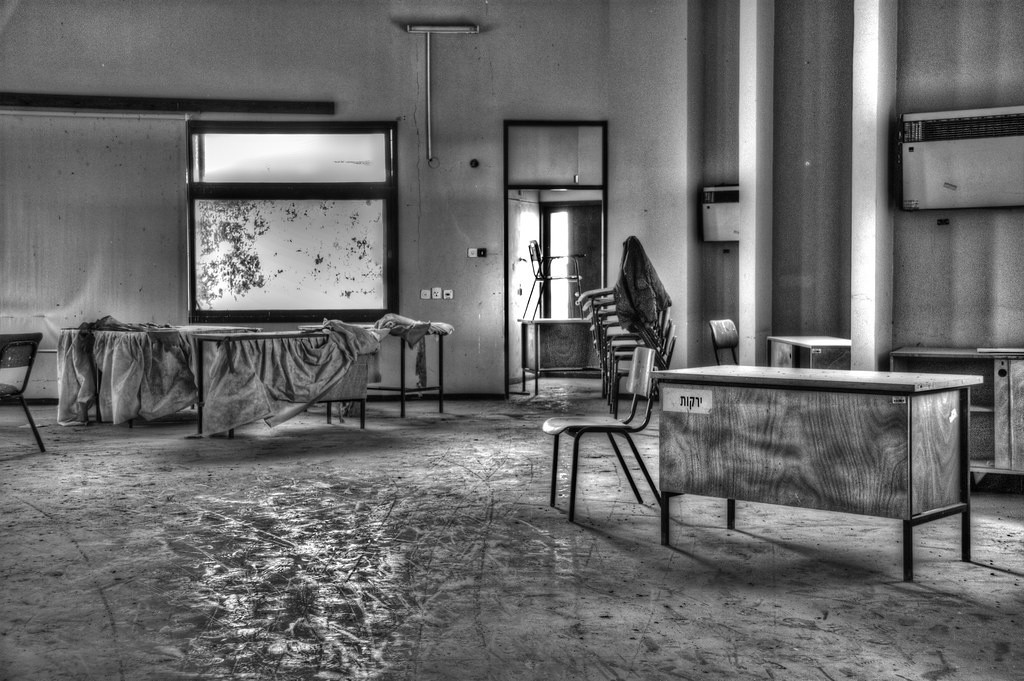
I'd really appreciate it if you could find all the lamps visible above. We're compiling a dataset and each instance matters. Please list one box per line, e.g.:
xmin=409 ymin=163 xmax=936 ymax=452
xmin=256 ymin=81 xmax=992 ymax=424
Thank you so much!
xmin=406 ymin=23 xmax=480 ymax=35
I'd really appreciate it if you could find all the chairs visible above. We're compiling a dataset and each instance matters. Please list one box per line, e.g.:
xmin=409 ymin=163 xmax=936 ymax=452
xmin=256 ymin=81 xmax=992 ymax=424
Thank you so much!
xmin=708 ymin=318 xmax=740 ymax=365
xmin=0 ymin=331 xmax=48 ymax=454
xmin=522 ymin=234 xmax=677 ymax=523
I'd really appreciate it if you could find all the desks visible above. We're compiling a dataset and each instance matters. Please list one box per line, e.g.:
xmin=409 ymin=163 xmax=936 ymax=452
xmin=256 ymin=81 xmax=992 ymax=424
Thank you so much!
xmin=182 ymin=330 xmax=383 ymax=439
xmin=297 ymin=320 xmax=452 ymax=419
xmin=516 ymin=318 xmax=601 ymax=395
xmin=54 ymin=327 xmax=265 ymax=429
xmin=888 ymin=345 xmax=1024 ymax=475
xmin=649 ymin=363 xmax=986 ymax=583
xmin=762 ymin=335 xmax=851 ymax=370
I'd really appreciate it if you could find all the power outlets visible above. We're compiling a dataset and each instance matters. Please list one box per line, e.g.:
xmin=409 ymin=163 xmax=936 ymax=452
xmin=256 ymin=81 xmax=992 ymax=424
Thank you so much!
xmin=431 ymin=287 xmax=442 ymax=299
